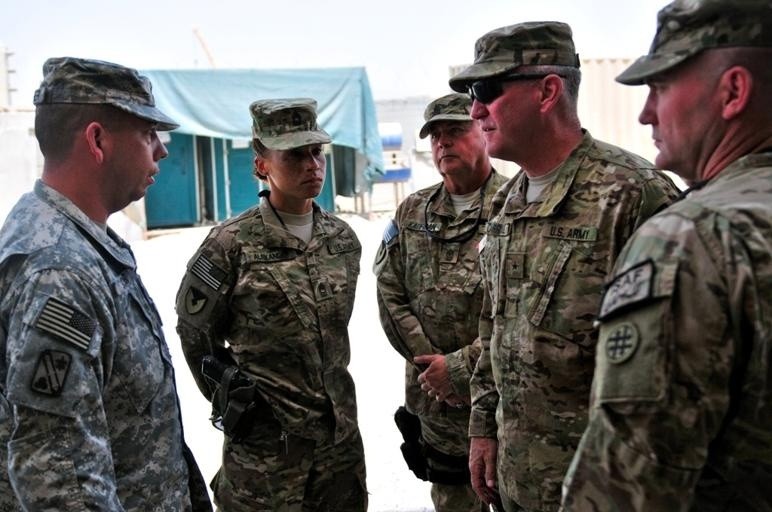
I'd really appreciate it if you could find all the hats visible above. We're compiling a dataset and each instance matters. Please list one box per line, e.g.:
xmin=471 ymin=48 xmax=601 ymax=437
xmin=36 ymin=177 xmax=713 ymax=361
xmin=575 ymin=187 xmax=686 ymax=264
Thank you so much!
xmin=416 ymin=91 xmax=476 ymax=139
xmin=248 ymin=96 xmax=336 ymax=152
xmin=611 ymin=0 xmax=772 ymax=89
xmin=446 ymin=18 xmax=583 ymax=95
xmin=32 ymin=54 xmax=182 ymax=133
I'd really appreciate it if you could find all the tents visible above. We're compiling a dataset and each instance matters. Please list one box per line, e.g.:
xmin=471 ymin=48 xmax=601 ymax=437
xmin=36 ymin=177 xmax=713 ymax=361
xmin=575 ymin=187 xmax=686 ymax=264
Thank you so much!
xmin=121 ymin=67 xmax=386 ymax=237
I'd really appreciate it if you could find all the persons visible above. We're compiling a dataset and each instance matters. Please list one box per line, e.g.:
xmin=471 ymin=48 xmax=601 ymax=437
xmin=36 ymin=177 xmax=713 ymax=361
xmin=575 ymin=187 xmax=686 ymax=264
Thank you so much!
xmin=373 ymin=93 xmax=510 ymax=511
xmin=174 ymin=98 xmax=369 ymax=511
xmin=0 ymin=58 xmax=215 ymax=512
xmin=447 ymin=21 xmax=683 ymax=511
xmin=558 ymin=0 xmax=771 ymax=511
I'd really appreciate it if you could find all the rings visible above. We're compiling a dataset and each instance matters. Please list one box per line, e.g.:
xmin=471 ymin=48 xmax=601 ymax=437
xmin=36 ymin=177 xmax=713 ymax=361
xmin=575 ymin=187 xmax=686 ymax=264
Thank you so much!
xmin=457 ymin=404 xmax=463 ymax=409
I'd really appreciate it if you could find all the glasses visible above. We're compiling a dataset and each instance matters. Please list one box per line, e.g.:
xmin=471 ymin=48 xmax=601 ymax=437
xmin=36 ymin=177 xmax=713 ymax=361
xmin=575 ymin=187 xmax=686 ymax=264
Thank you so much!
xmin=466 ymin=73 xmax=569 ymax=105
xmin=422 ymin=192 xmax=484 ymax=245
